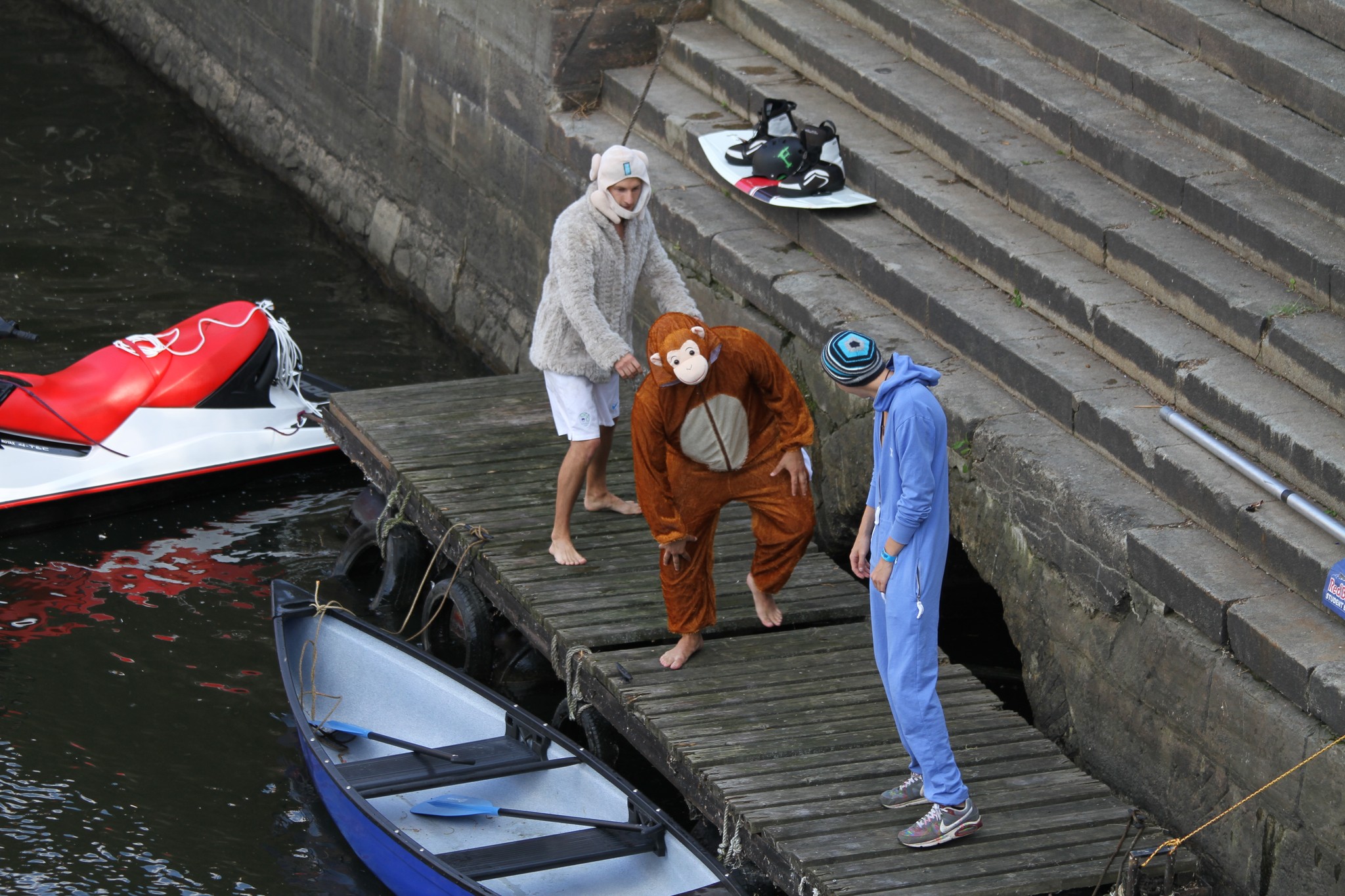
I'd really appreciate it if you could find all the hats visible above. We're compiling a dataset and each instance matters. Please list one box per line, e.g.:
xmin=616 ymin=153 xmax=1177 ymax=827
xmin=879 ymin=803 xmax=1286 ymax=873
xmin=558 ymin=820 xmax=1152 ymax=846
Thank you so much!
xmin=821 ymin=328 xmax=886 ymax=386
xmin=587 ymin=145 xmax=652 ymax=226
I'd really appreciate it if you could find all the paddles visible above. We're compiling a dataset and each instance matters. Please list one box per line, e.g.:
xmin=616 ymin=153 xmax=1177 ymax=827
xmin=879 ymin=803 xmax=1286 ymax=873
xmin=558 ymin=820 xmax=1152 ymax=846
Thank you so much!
xmin=312 ymin=714 xmax=474 ymax=767
xmin=410 ymin=792 xmax=662 ymax=837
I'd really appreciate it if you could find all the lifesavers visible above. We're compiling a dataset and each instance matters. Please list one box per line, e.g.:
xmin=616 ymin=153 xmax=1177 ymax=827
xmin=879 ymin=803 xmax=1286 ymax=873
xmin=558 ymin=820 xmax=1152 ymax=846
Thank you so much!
xmin=547 ymin=695 xmax=615 ymax=765
xmin=419 ymin=572 xmax=493 ymax=682
xmin=319 ymin=514 xmax=417 ymax=619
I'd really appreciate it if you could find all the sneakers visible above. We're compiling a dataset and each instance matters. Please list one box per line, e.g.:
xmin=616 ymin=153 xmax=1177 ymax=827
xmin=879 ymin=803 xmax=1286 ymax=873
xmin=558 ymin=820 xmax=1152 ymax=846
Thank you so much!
xmin=724 ymin=99 xmax=798 ymax=166
xmin=777 ymin=123 xmax=845 ymax=198
xmin=897 ymin=798 xmax=984 ymax=848
xmin=880 ymin=772 xmax=932 ymax=810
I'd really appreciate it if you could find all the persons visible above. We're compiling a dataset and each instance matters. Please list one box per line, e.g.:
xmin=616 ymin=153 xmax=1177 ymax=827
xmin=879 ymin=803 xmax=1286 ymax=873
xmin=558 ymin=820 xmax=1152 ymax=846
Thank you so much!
xmin=529 ymin=145 xmax=702 ymax=565
xmin=818 ymin=331 xmax=984 ymax=849
xmin=630 ymin=311 xmax=816 ymax=668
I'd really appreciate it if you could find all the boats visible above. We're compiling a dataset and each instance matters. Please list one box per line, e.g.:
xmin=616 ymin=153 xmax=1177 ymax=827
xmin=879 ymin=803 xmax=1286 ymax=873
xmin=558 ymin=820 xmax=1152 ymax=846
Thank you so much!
xmin=270 ymin=578 xmax=753 ymax=896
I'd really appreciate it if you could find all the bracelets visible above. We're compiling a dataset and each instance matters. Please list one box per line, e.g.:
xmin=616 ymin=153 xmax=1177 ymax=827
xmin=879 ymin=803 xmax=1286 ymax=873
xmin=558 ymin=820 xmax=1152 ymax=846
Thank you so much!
xmin=881 ymin=548 xmax=897 ymax=563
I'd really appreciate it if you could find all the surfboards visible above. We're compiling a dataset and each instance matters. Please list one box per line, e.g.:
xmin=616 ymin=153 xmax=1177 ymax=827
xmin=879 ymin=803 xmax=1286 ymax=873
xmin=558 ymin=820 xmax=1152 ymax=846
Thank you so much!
xmin=698 ymin=98 xmax=880 ymax=214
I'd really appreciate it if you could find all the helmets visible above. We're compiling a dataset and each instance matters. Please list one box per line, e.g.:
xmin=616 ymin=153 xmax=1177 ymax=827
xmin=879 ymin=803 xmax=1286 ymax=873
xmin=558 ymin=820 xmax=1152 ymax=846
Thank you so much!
xmin=751 ymin=135 xmax=807 ymax=179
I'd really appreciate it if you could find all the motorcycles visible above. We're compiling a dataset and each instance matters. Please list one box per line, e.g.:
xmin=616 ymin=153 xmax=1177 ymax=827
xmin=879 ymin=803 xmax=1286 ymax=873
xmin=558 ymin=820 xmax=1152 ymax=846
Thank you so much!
xmin=0 ymin=298 xmax=350 ymax=520
